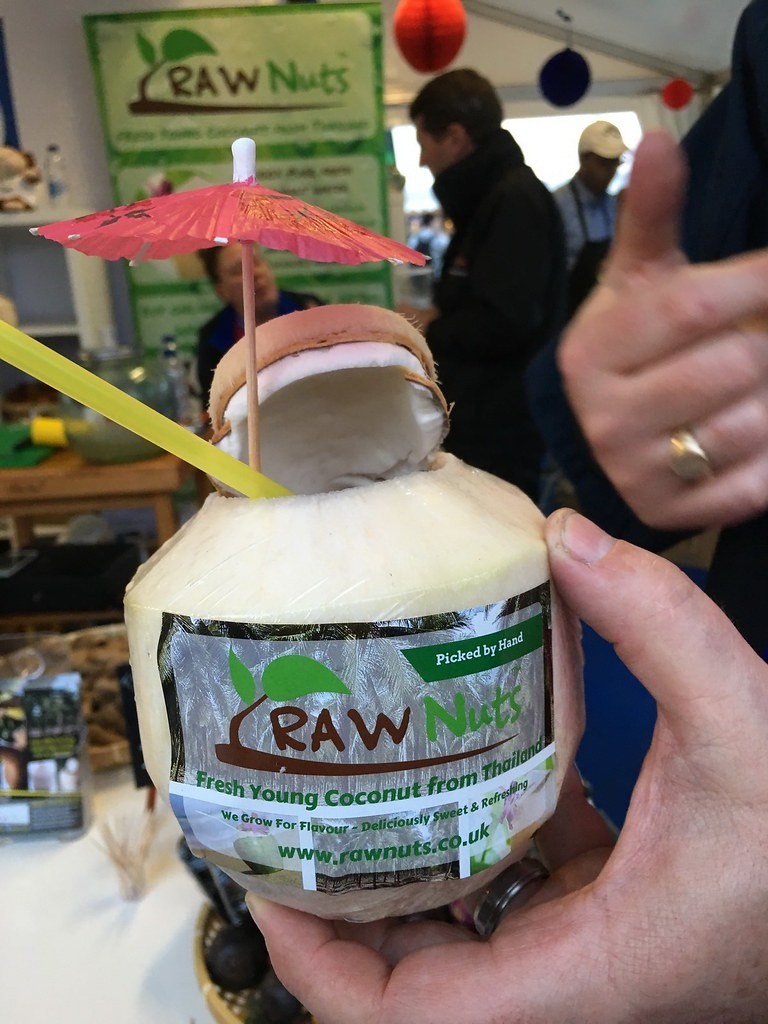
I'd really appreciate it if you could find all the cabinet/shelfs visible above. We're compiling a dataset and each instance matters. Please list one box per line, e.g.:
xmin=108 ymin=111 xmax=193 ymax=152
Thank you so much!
xmin=1 ymin=208 xmax=117 ymax=350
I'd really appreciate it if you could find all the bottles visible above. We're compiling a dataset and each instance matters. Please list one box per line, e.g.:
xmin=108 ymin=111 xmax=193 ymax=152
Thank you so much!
xmin=59 ymin=347 xmax=176 ymax=461
xmin=44 ymin=144 xmax=69 ymax=206
xmin=162 ymin=335 xmax=193 ymax=426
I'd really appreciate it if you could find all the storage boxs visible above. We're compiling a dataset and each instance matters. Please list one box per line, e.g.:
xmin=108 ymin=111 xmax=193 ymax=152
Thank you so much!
xmin=0 ymin=670 xmax=87 ymax=837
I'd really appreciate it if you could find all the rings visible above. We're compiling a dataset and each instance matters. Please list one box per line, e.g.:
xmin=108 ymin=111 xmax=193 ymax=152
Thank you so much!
xmin=475 ymin=860 xmax=551 ymax=939
xmin=671 ymin=427 xmax=715 ymax=483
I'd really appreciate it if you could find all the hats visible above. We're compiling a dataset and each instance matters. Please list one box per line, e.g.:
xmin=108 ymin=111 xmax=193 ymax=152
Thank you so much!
xmin=579 ymin=122 xmax=632 ymax=159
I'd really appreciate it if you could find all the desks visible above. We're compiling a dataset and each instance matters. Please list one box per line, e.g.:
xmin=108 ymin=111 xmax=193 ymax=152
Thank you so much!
xmin=0 ymin=420 xmax=209 ymax=623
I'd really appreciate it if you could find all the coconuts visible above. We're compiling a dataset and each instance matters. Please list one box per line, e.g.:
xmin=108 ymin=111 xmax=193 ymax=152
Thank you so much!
xmin=123 ymin=302 xmax=586 ymax=925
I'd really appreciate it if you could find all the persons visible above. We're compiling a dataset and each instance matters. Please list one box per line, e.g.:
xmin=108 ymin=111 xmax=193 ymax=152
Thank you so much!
xmin=408 ymin=214 xmax=449 ymax=276
xmin=553 ymin=122 xmax=634 ymax=295
xmin=191 ymin=242 xmax=325 ymax=419
xmin=405 ymin=67 xmax=571 ymax=485
xmin=244 ymin=0 xmax=767 ymax=1024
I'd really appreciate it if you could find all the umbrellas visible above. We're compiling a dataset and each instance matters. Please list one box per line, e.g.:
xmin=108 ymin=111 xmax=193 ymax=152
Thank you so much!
xmin=29 ymin=138 xmax=432 ymax=472
